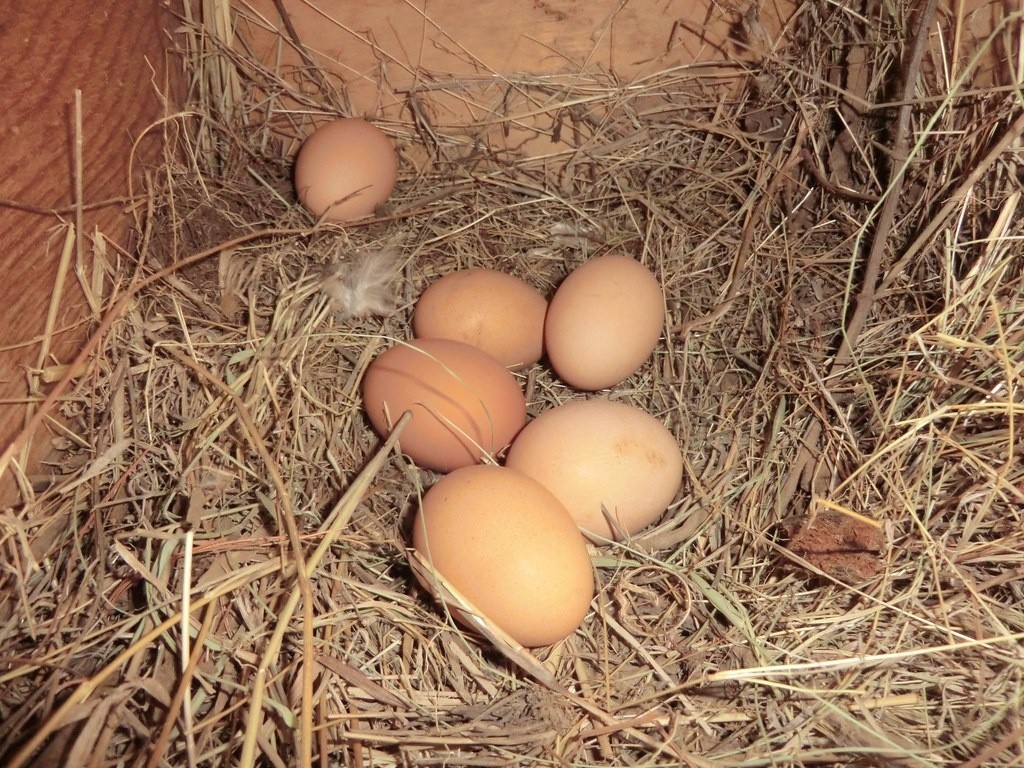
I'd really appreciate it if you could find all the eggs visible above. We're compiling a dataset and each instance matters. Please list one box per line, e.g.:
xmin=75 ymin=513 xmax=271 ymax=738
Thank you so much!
xmin=296 ymin=117 xmax=396 ymax=224
xmin=363 ymin=256 xmax=683 ymax=648
